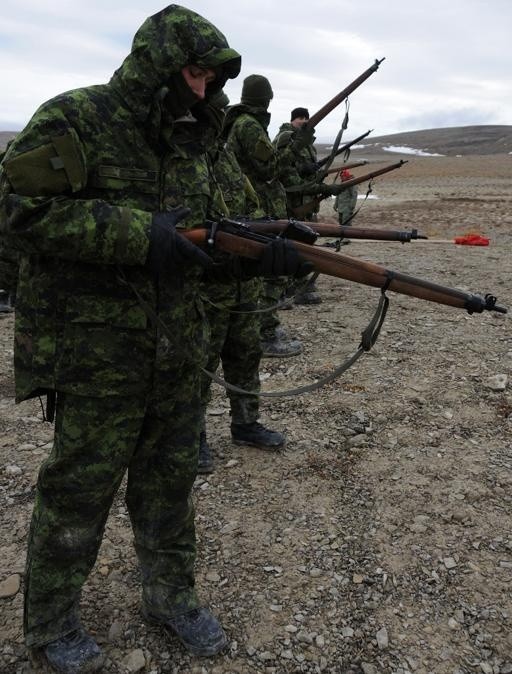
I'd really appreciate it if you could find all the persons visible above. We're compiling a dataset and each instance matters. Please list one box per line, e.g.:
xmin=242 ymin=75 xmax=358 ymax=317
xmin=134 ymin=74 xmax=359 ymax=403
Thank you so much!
xmin=332 ymin=168 xmax=358 ymax=244
xmin=1 ymin=4 xmax=313 ymax=674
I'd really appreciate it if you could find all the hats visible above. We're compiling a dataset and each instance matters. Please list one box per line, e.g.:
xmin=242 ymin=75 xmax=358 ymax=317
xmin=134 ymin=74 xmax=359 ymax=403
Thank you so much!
xmin=241 ymin=74 xmax=274 ymax=105
xmin=290 ymin=107 xmax=310 ymax=123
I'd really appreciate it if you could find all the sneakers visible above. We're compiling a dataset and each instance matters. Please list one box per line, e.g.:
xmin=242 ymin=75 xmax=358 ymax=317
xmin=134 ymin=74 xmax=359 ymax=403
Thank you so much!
xmin=294 ymin=294 xmax=323 ymax=305
xmin=42 ymin=624 xmax=106 ymax=674
xmin=259 ymin=327 xmax=305 ymax=358
xmin=198 ymin=429 xmax=216 ymax=477
xmin=161 ymin=604 xmax=228 ymax=661
xmin=276 ymin=297 xmax=292 ymax=311
xmin=229 ymin=421 xmax=285 ymax=453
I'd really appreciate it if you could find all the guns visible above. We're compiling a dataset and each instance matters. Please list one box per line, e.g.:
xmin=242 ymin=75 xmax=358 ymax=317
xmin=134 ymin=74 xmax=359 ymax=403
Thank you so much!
xmin=224 ymin=219 xmax=429 ymax=244
xmin=315 ymin=130 xmax=374 ymax=169
xmin=295 ymin=160 xmax=408 ymax=219
xmin=304 ymin=56 xmax=385 ymax=130
xmin=181 ymin=219 xmax=507 ymax=317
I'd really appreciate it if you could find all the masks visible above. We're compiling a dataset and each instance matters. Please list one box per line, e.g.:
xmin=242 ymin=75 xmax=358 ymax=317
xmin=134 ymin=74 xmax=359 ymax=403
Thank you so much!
xmin=159 ymin=70 xmax=201 ymax=111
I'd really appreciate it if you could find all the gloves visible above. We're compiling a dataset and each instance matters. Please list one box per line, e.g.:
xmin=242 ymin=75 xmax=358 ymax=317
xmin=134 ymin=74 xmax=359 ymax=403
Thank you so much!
xmin=292 ymin=122 xmax=316 ymax=149
xmin=328 ymin=183 xmax=345 ymax=197
xmin=244 ymin=237 xmax=316 ymax=282
xmin=302 ymin=162 xmax=320 ymax=177
xmin=142 ymin=205 xmax=216 ymax=280
